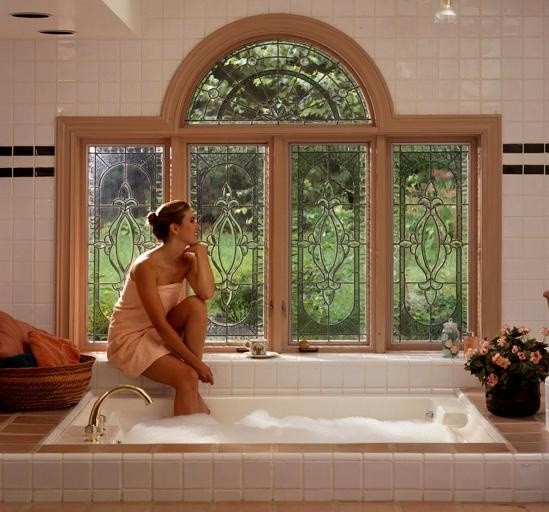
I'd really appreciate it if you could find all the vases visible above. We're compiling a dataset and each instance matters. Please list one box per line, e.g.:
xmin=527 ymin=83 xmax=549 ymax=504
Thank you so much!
xmin=484 ymin=371 xmax=541 ymax=419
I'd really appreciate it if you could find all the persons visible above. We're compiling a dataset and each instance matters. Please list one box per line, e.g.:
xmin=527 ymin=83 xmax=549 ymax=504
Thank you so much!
xmin=105 ymin=200 xmax=215 ymax=415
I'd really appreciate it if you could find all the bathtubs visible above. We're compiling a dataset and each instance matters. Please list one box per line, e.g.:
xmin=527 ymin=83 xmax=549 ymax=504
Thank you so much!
xmin=39 ymin=385 xmax=509 ymax=444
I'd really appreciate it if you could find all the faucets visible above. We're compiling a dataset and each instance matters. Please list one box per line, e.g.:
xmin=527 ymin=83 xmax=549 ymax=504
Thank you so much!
xmin=87 ymin=383 xmax=152 ymax=429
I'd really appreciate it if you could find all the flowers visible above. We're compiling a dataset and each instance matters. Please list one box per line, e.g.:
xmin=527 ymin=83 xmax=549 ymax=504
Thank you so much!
xmin=459 ymin=324 xmax=549 ymax=400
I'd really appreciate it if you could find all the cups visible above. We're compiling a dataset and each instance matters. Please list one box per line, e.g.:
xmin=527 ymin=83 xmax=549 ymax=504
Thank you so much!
xmin=250 ymin=339 xmax=268 ymax=354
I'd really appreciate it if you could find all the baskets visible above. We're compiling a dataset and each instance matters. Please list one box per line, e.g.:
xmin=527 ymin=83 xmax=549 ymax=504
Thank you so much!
xmin=0 ymin=354 xmax=96 ymax=409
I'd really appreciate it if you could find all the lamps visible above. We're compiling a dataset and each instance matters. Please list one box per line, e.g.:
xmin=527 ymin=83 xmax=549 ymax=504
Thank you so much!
xmin=431 ymin=1 xmax=460 ymax=25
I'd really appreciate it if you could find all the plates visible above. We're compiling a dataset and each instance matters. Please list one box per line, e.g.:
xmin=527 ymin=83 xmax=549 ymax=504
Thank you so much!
xmin=243 ymin=350 xmax=280 ymax=358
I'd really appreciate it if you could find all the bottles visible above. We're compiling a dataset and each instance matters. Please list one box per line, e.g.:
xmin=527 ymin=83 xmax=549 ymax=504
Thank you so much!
xmin=441 ymin=317 xmax=460 ymax=357
